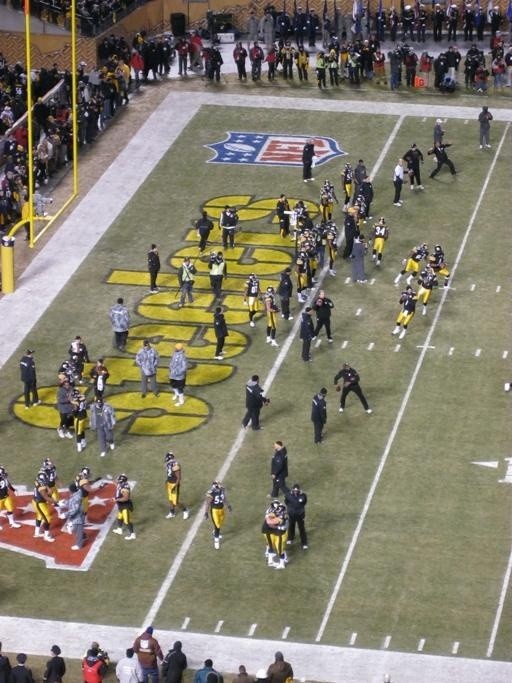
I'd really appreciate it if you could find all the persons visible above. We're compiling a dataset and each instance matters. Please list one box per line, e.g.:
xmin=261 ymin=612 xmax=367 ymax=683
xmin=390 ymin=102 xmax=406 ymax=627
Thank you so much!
xmin=478 ymin=105 xmax=493 ymax=149
xmin=58 ymin=466 xmax=104 ymax=550
xmin=265 ymin=39 xmax=310 ymax=81
xmin=387 ymin=1 xmax=486 ymax=42
xmin=433 ymin=118 xmax=446 ymax=148
xmin=162 ymin=452 xmax=190 ymax=519
xmin=266 ymin=442 xmax=291 ymax=499
xmin=208 ymin=251 xmax=227 ymax=299
xmin=116 ymin=648 xmax=144 ymax=683
xmin=243 ymin=272 xmax=263 ymax=326
xmin=263 ymin=499 xmax=289 ymax=568
xmin=284 ymin=485 xmax=308 ymax=549
xmin=387 ymin=42 xmax=418 ymax=91
xmin=220 ymin=205 xmax=236 ymax=249
xmin=161 ymin=640 xmax=187 ymax=683
xmin=135 ymin=339 xmax=160 ymax=399
xmin=132 ymin=627 xmax=163 ymax=683
xmin=213 ymin=307 xmax=229 ymax=359
xmin=314 ymin=5 xmax=383 ymax=90
xmin=0 ymin=35 xmax=131 ymax=237
xmin=489 ymin=40 xmax=512 ymax=89
xmin=192 ymin=211 xmax=214 ymax=254
xmin=241 ymin=375 xmax=269 ymax=432
xmin=194 ymin=659 xmax=223 ymax=683
xmin=311 ymin=388 xmax=327 ymax=444
xmin=250 ymin=41 xmax=264 ymax=82
xmin=489 ymin=5 xmax=507 ymax=39
xmin=131 ymin=28 xmax=224 ymax=93
xmin=19 ymin=349 xmax=41 ymax=410
xmin=42 ymin=645 xmax=65 ymax=683
xmin=0 ymin=464 xmax=22 ymax=529
xmin=247 ymin=7 xmax=314 ymax=46
xmin=168 ymin=343 xmax=187 ymax=409
xmin=232 ymin=666 xmax=257 ymax=683
xmin=416 ymin=264 xmax=437 ymax=315
xmin=312 ymin=290 xmax=334 ymax=343
xmin=300 ymin=307 xmax=315 ymax=360
xmin=340 ymin=159 xmax=390 ymax=283
xmin=427 ymin=244 xmax=450 ymax=290
xmin=0 ymin=640 xmax=11 ymax=683
xmin=428 ymin=140 xmax=457 ymax=179
xmin=89 ymin=397 xmax=116 ymax=458
xmin=90 ymin=359 xmax=110 ymax=408
xmin=433 ymin=47 xmax=462 ymax=92
xmin=393 ymin=158 xmax=415 ymax=207
xmin=266 ymin=652 xmax=293 ymax=683
xmin=402 ymin=144 xmax=425 ymax=190
xmin=277 ymin=195 xmax=290 ymax=235
xmin=464 ymin=44 xmax=491 ymax=92
xmin=264 ymin=286 xmax=280 ymax=347
xmin=83 ymin=642 xmax=108 ymax=683
xmin=276 ymin=268 xmax=294 ymax=322
xmin=147 ymin=244 xmax=161 ymax=293
xmin=109 ymin=475 xmax=136 ymax=540
xmin=109 ymin=298 xmax=131 ymax=352
xmin=292 ymin=179 xmax=340 ymax=303
xmin=334 ymin=362 xmax=373 ymax=414
xmin=31 ymin=455 xmax=60 ymax=543
xmin=203 ymin=480 xmax=232 ymax=550
xmin=391 ymin=285 xmax=418 ymax=339
xmin=8 ymin=653 xmax=35 ymax=683
xmin=232 ymin=41 xmax=248 ymax=81
xmin=56 ymin=336 xmax=89 ymax=453
xmin=302 ymin=140 xmax=319 ymax=182
xmin=420 ymin=50 xmax=432 ymax=88
xmin=178 ymin=256 xmax=197 ymax=307
xmin=393 ymin=243 xmax=429 ymax=286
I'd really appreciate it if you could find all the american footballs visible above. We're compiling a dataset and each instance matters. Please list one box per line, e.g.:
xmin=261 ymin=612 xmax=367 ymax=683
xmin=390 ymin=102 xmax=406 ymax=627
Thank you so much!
xmin=336 ymin=384 xmax=341 ymax=392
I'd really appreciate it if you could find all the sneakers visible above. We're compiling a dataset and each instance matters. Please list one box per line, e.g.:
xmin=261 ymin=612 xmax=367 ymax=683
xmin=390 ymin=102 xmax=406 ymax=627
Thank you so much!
xmin=406 ymin=278 xmax=411 ymax=285
xmin=112 ymin=527 xmax=123 ymax=535
xmin=77 ymin=444 xmax=82 ymax=453
xmin=271 ymin=342 xmax=280 ymax=348
xmin=339 ymin=408 xmax=344 ymax=412
xmin=399 ymin=200 xmax=404 ymax=204
xmin=110 ymin=443 xmax=115 ymax=450
xmin=422 ymin=309 xmax=427 ymax=315
xmin=141 ymin=394 xmax=148 ymax=399
xmin=71 ymin=545 xmax=81 ymax=550
xmin=173 ymin=395 xmax=177 ymax=400
xmin=33 ymin=400 xmax=42 ymax=408
xmin=394 ymin=202 xmax=401 ymax=208
xmin=399 ymin=331 xmax=406 ymax=339
xmin=8 ymin=523 xmax=22 ymax=529
xmin=214 ymin=356 xmax=224 ymax=360
xmin=174 ymin=402 xmax=186 ymax=407
xmin=43 ymin=535 xmax=56 ymax=542
xmin=250 ymin=321 xmax=256 ymax=327
xmin=213 ymin=530 xmax=223 ymax=539
xmin=218 ymin=351 xmax=227 ymax=354
xmin=214 ymin=537 xmax=219 ymax=550
xmin=33 ymin=532 xmax=43 ymax=539
xmin=393 ymin=279 xmax=399 ymax=284
xmin=100 ymin=450 xmax=106 ymax=457
xmin=154 ymin=393 xmax=160 ymax=397
xmin=284 ymin=552 xmax=289 ymax=563
xmin=266 ymin=338 xmax=270 ymax=343
xmin=57 ymin=427 xmax=64 ymax=439
xmin=268 ymin=552 xmax=275 ymax=566
xmin=313 ymin=338 xmax=317 ymax=340
xmin=64 ymin=429 xmax=74 ymax=440
xmin=25 ymin=404 xmax=31 ymax=409
xmin=165 ymin=513 xmax=176 ymax=519
xmin=124 ymin=533 xmax=136 ymax=541
xmin=183 ymin=507 xmax=190 ymax=519
xmin=329 ymin=338 xmax=334 ymax=343
xmin=365 ymin=409 xmax=373 ymax=415
xmin=302 ymin=545 xmax=308 ymax=550
xmin=286 ymin=540 xmax=291 ymax=544
xmin=276 ymin=558 xmax=285 ymax=569
xmin=81 ymin=439 xmax=86 ymax=448
xmin=392 ymin=329 xmax=401 ymax=335
xmin=265 ymin=544 xmax=269 ymax=557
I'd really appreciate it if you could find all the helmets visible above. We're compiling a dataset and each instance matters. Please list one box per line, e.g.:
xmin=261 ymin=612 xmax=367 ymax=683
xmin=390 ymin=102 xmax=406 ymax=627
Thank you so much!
xmin=82 ymin=468 xmax=89 ymax=478
xmin=211 ymin=482 xmax=218 ymax=490
xmin=272 ymin=502 xmax=278 ymax=509
xmin=267 ymin=287 xmax=273 ymax=294
xmin=165 ymin=452 xmax=174 ymax=462
xmin=249 ymin=273 xmax=255 ymax=280
xmin=276 ymin=506 xmax=285 ymax=513
xmin=37 ymin=473 xmax=46 ymax=483
xmin=118 ymin=474 xmax=128 ymax=483
